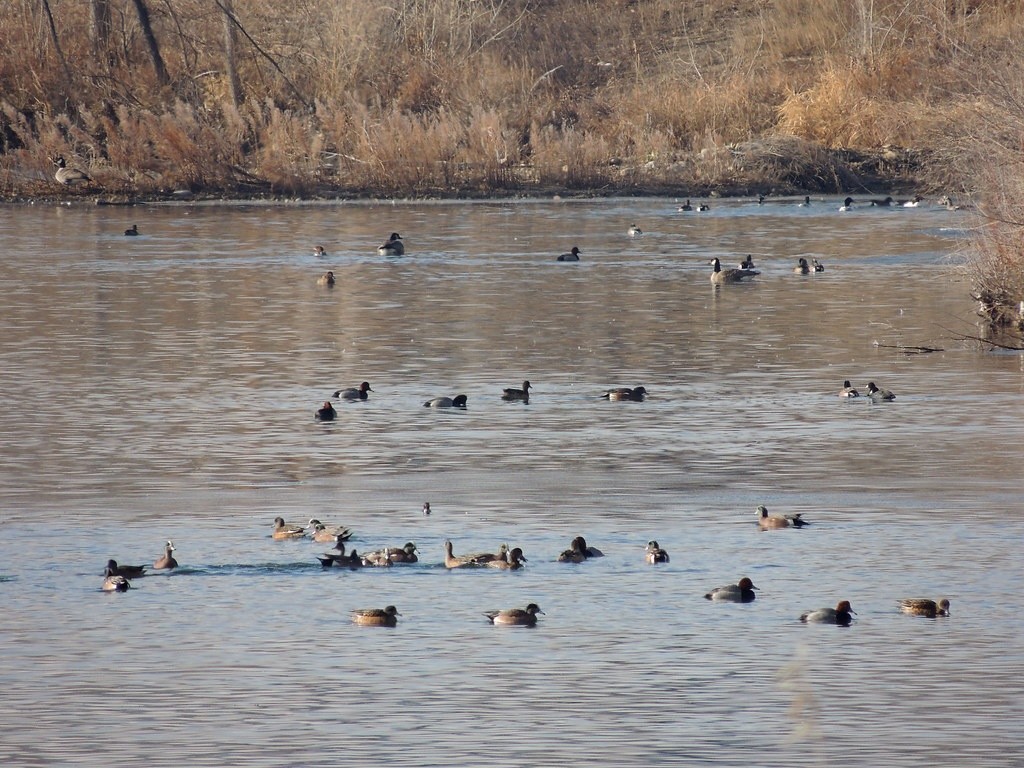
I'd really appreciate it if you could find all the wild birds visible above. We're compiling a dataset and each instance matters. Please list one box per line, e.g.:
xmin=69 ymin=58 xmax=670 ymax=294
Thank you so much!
xmin=101 ymin=568 xmax=130 ymax=590
xmin=483 ymin=602 xmax=545 ymax=626
xmin=703 ymin=577 xmax=760 ymax=602
xmin=799 ymin=600 xmax=858 ymax=627
xmin=272 ymin=517 xmax=421 ymax=567
xmin=315 ymin=382 xmax=374 ymax=421
xmin=754 ymin=505 xmax=808 ymax=528
xmin=307 ymin=191 xmax=970 ymax=285
xmin=894 ymin=597 xmax=952 ymax=618
xmin=421 ymin=500 xmax=670 ymax=570
xmin=603 ymin=386 xmax=651 ymax=402
xmin=153 ymin=541 xmax=178 ymax=569
xmin=841 ymin=379 xmax=859 ymax=399
xmin=123 ymin=223 xmax=139 ymax=238
xmin=864 ymin=383 xmax=898 ymax=402
xmin=350 ymin=606 xmax=403 ymax=628
xmin=502 ymin=380 xmax=533 ymax=399
xmin=318 ymin=270 xmax=335 ymax=288
xmin=423 ymin=392 xmax=466 ymax=409
xmin=104 ymin=559 xmax=147 ymax=578
